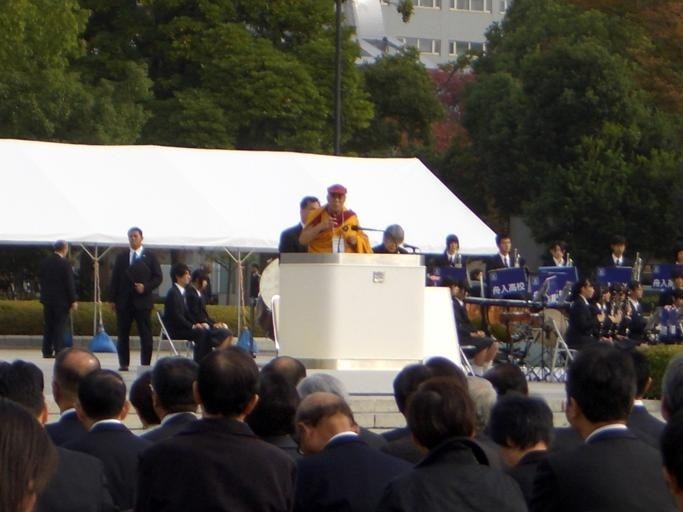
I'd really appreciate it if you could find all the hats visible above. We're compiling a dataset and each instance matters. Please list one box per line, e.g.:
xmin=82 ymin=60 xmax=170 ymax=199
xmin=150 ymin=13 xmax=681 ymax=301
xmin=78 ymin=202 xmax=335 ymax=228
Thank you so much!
xmin=328 ymin=185 xmax=347 ymax=195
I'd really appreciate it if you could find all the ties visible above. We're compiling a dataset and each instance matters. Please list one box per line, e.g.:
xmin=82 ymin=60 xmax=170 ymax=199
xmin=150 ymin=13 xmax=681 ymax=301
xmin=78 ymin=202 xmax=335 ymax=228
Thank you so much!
xmin=132 ymin=252 xmax=137 ymax=265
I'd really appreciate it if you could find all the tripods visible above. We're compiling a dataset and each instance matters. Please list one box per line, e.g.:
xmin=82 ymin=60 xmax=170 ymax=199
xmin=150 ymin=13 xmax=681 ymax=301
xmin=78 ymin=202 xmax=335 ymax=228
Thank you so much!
xmin=505 ymin=304 xmax=569 ymax=381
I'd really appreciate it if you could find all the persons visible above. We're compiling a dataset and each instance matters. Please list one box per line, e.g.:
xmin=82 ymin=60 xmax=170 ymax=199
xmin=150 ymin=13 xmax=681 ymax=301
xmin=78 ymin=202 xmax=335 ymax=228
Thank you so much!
xmin=38 ymin=239 xmax=79 ymax=358
xmin=160 ymin=261 xmax=233 ymax=363
xmin=248 ymin=263 xmax=261 ymax=297
xmin=426 ymin=234 xmax=683 ymax=376
xmin=108 ymin=227 xmax=163 ymax=372
xmin=279 ymin=184 xmax=409 ymax=256
xmin=0 ymin=340 xmax=683 ymax=512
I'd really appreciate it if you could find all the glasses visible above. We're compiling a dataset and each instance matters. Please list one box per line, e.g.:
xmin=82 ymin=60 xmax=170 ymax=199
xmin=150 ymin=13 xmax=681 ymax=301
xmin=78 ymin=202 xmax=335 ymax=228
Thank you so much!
xmin=297 ymin=428 xmax=306 ymax=457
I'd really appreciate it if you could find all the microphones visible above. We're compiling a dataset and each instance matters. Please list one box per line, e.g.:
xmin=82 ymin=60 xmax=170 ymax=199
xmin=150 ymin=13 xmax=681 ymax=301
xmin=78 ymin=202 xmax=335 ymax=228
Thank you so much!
xmin=352 ymin=225 xmax=398 ymax=253
xmin=403 ymin=243 xmax=419 ymax=249
